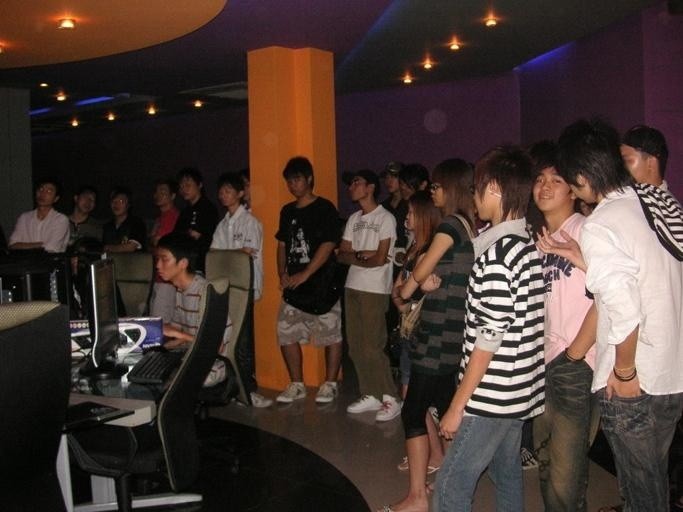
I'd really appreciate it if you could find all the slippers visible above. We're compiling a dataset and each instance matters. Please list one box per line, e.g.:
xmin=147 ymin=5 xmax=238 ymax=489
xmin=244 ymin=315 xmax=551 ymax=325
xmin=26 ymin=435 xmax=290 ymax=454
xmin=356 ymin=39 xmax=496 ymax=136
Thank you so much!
xmin=399 ymin=455 xmax=441 ymax=474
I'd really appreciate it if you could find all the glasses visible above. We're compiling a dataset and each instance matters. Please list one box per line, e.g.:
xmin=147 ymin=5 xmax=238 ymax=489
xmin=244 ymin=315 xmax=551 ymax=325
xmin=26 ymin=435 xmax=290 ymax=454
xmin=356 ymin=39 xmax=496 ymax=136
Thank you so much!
xmin=430 ymin=183 xmax=446 ymax=191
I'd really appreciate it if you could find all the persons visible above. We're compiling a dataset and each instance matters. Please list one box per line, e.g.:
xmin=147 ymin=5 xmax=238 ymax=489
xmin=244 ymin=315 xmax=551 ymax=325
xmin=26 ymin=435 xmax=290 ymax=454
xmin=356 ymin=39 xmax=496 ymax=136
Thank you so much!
xmin=1 ymin=115 xmax=682 ymax=511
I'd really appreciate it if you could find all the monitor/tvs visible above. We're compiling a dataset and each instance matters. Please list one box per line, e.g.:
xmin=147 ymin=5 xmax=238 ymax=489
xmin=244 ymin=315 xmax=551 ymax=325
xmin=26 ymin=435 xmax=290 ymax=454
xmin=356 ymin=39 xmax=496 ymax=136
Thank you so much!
xmin=78 ymin=257 xmax=129 ymax=380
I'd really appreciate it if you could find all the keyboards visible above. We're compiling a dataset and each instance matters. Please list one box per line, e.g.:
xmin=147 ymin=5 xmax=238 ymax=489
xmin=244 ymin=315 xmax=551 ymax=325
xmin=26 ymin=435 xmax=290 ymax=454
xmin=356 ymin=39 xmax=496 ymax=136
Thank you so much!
xmin=127 ymin=350 xmax=176 ymax=384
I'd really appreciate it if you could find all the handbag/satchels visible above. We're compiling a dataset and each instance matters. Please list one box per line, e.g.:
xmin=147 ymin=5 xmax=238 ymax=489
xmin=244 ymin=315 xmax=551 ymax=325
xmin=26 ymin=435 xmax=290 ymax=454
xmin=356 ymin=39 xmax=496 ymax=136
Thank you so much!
xmin=398 ymin=294 xmax=426 ymax=341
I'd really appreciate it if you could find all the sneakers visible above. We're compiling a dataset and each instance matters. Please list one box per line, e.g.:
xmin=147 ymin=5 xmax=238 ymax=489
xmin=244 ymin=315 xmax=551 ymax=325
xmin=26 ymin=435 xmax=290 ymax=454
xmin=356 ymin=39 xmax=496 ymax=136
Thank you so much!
xmin=375 ymin=392 xmax=401 ymax=422
xmin=315 ymin=382 xmax=339 ymax=403
xmin=520 ymin=449 xmax=542 ymax=470
xmin=276 ymin=383 xmax=306 ymax=403
xmin=231 ymin=392 xmax=272 ymax=408
xmin=346 ymin=394 xmax=383 ymax=414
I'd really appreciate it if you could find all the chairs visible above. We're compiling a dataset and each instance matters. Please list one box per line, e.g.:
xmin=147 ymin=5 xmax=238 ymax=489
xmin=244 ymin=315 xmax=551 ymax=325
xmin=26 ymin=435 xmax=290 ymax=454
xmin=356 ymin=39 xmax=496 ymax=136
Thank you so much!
xmin=70 ymin=275 xmax=227 ymax=512
xmin=3 ymin=300 xmax=78 ymax=510
xmin=108 ymin=250 xmax=155 ymax=316
xmin=182 ymin=248 xmax=257 ymax=401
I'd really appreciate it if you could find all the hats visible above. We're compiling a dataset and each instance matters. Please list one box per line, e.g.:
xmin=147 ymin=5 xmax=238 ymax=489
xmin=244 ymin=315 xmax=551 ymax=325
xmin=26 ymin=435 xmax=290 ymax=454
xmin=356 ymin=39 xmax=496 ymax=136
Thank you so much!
xmin=622 ymin=123 xmax=668 ymax=160
xmin=380 ymin=159 xmax=402 ymax=176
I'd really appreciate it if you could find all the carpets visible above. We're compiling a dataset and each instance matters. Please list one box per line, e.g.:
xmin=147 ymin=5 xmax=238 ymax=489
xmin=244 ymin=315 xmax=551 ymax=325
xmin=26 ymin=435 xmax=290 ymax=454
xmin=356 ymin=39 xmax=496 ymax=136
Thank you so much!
xmin=66 ymin=415 xmax=371 ymax=512
